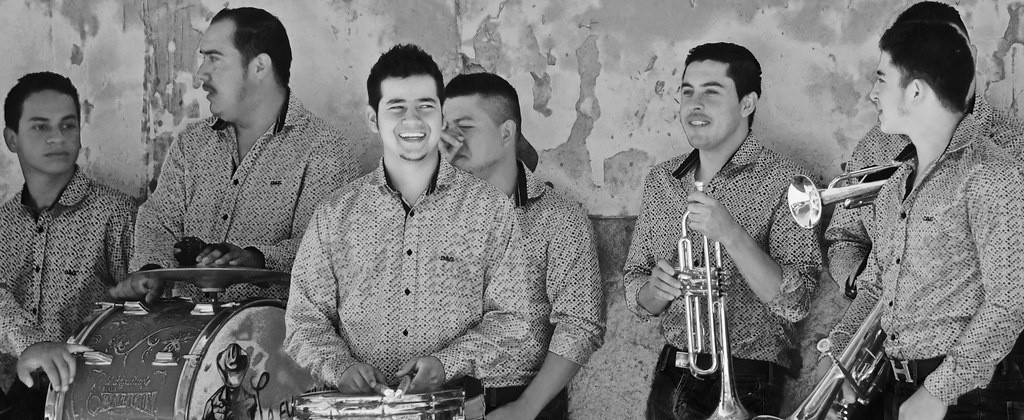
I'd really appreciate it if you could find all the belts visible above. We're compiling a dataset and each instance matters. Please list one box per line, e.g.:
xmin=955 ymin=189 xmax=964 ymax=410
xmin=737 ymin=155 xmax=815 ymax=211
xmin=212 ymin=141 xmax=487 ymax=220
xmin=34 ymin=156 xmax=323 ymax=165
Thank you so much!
xmin=885 ymin=356 xmax=946 ymax=383
xmin=482 ymin=385 xmax=528 ymax=407
xmin=659 ymin=345 xmax=768 ymax=377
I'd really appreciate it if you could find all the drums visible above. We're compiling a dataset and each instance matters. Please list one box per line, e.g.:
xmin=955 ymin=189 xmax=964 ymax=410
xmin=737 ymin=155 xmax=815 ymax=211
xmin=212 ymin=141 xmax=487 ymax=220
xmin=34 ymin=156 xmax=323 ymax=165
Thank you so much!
xmin=296 ymin=385 xmax=467 ymax=420
xmin=44 ymin=296 xmax=315 ymax=420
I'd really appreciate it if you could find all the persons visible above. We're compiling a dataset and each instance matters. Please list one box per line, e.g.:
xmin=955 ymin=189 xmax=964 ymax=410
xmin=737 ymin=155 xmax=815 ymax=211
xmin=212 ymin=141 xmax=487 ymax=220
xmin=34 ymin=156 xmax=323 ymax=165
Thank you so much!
xmin=125 ymin=7 xmax=372 ymax=306
xmin=823 ymin=1 xmax=1024 ymax=420
xmin=621 ymin=40 xmax=822 ymax=420
xmin=282 ymin=43 xmax=532 ymax=420
xmin=435 ymin=72 xmax=605 ymax=420
xmin=0 ymin=72 xmax=141 ymax=420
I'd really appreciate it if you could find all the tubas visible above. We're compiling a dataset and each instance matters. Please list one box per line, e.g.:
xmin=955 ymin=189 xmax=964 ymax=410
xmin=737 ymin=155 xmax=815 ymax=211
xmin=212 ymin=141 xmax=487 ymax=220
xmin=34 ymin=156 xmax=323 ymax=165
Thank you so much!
xmin=751 ymin=294 xmax=891 ymax=420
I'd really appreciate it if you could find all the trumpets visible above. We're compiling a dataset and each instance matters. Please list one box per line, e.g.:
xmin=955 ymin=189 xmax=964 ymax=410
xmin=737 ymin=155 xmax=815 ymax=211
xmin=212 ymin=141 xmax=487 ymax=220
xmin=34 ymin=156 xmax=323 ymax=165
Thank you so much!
xmin=673 ymin=180 xmax=756 ymax=420
xmin=786 ymin=161 xmax=905 ymax=230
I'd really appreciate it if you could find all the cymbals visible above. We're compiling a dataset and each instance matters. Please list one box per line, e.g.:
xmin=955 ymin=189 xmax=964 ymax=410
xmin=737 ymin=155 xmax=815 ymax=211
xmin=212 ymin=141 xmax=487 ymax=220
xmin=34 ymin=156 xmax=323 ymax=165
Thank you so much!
xmin=133 ymin=266 xmax=291 ymax=294
